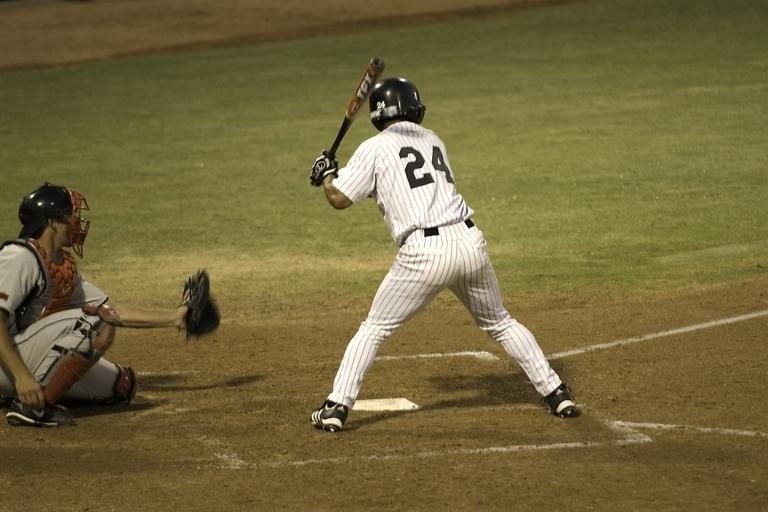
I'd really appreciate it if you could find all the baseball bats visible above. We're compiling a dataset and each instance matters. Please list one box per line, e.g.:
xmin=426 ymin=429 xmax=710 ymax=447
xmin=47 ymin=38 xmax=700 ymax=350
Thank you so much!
xmin=311 ymin=57 xmax=385 ymax=187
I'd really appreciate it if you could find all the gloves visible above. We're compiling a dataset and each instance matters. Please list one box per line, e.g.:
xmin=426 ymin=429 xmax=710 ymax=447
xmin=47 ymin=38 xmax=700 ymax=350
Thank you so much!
xmin=310 ymin=149 xmax=339 ymax=186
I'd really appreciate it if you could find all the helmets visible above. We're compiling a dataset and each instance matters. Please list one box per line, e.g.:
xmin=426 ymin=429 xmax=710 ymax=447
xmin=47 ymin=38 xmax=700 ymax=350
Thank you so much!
xmin=17 ymin=180 xmax=89 ymax=258
xmin=369 ymin=78 xmax=426 ymax=131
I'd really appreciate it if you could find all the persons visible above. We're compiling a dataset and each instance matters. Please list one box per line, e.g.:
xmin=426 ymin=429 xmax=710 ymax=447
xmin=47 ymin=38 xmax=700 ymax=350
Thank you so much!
xmin=0 ymin=183 xmax=193 ymax=426
xmin=309 ymin=77 xmax=575 ymax=432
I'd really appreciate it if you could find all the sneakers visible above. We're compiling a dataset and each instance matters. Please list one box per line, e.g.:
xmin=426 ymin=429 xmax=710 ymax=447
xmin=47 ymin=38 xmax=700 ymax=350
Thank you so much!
xmin=310 ymin=401 xmax=348 ymax=431
xmin=6 ymin=397 xmax=60 ymax=427
xmin=545 ymin=386 xmax=576 ymax=418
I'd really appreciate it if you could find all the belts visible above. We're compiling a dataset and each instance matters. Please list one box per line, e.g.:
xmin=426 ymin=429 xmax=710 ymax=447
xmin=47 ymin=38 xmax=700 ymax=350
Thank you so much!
xmin=424 ymin=218 xmax=474 ymax=236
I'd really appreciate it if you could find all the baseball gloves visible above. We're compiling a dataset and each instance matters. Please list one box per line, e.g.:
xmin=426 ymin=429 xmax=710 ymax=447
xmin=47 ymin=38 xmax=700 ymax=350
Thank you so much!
xmin=174 ymin=268 xmax=220 ymax=342
